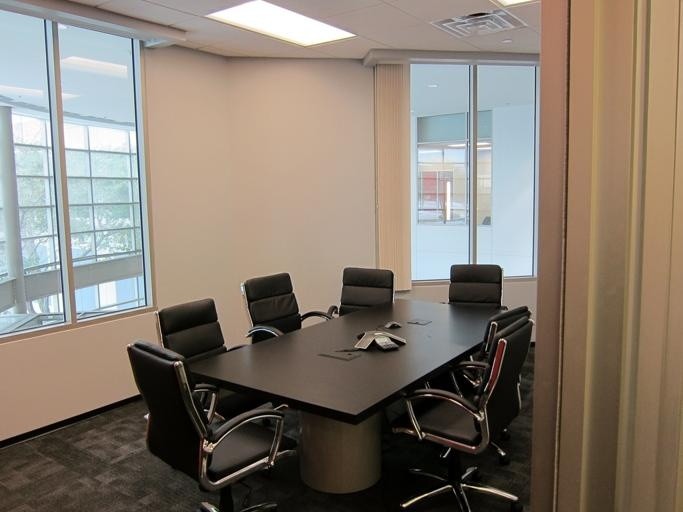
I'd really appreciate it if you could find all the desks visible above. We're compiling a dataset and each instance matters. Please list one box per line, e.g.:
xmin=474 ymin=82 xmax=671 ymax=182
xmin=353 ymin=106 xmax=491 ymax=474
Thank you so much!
xmin=188 ymin=298 xmax=509 ymax=495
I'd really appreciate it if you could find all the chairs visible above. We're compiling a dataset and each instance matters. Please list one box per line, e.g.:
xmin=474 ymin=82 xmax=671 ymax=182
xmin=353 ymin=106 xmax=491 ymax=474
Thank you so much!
xmin=126 ymin=339 xmax=300 ymax=512
xmin=153 ymin=298 xmax=288 ymax=432
xmin=447 ymin=265 xmax=503 ymax=307
xmin=326 ymin=266 xmax=394 ymax=318
xmin=241 ymin=272 xmax=333 ymax=337
xmin=388 ymin=316 xmax=535 ymax=511
xmin=433 ymin=306 xmax=530 ymax=462
